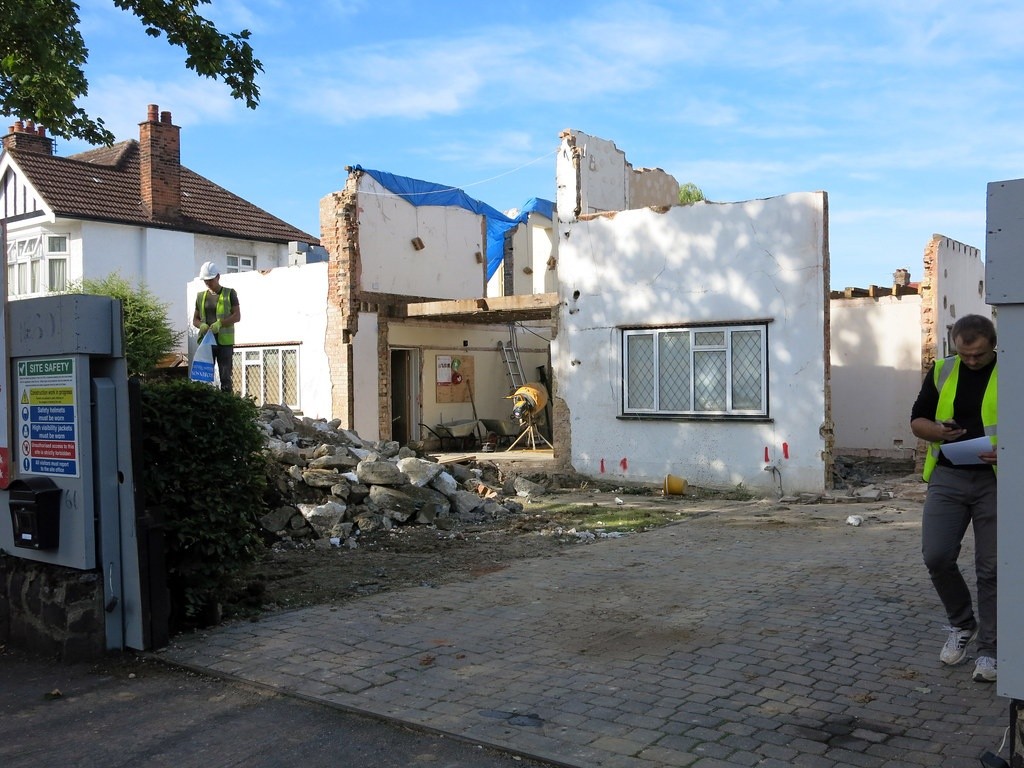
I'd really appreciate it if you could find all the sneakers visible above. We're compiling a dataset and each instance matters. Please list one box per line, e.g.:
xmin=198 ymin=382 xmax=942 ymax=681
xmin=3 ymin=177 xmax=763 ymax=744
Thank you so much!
xmin=939 ymin=621 xmax=981 ymax=665
xmin=972 ymin=657 xmax=998 ymax=683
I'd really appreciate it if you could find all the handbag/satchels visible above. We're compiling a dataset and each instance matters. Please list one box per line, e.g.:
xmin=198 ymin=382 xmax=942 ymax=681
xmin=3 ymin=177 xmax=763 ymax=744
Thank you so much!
xmin=190 ymin=330 xmax=217 ymax=383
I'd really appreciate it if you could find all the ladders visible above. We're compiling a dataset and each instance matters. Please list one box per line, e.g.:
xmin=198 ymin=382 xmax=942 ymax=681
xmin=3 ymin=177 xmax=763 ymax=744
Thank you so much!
xmin=497 ymin=340 xmax=542 ymax=447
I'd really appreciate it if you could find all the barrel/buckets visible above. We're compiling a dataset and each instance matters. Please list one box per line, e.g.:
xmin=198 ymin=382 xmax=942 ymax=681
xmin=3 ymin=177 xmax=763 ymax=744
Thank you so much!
xmin=664 ymin=474 xmax=688 ymax=495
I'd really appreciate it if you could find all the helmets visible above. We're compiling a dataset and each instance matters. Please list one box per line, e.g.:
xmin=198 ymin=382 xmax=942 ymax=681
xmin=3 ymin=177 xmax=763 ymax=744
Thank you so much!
xmin=199 ymin=262 xmax=218 ymax=280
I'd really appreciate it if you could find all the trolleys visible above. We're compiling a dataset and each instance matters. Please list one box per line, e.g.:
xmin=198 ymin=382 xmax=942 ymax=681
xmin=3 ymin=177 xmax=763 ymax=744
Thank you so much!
xmin=417 ymin=418 xmax=479 ymax=453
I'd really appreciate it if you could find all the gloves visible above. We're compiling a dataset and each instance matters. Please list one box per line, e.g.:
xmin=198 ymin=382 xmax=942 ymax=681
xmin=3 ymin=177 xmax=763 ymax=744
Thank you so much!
xmin=210 ymin=320 xmax=222 ymax=333
xmin=199 ymin=323 xmax=210 ymax=336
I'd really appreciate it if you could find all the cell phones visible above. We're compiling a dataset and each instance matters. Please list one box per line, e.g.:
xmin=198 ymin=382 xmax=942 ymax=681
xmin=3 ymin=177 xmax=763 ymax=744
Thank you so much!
xmin=942 ymin=423 xmax=962 ymax=430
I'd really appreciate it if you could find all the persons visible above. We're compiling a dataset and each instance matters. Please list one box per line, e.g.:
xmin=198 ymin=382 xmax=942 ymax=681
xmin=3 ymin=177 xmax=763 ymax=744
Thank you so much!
xmin=193 ymin=261 xmax=240 ymax=393
xmin=910 ymin=315 xmax=998 ymax=682
